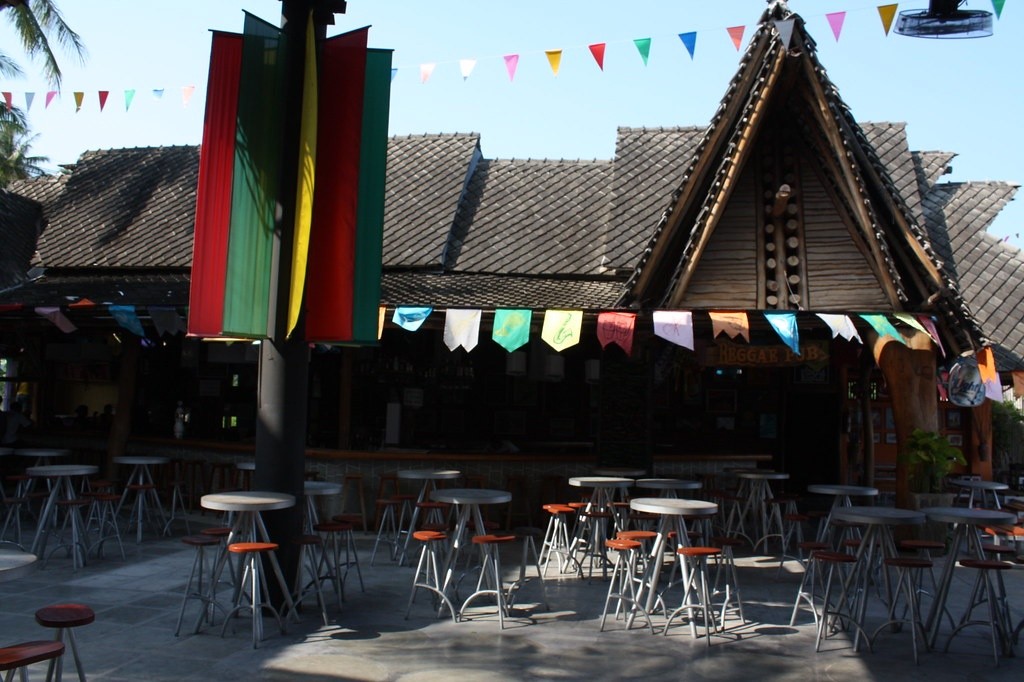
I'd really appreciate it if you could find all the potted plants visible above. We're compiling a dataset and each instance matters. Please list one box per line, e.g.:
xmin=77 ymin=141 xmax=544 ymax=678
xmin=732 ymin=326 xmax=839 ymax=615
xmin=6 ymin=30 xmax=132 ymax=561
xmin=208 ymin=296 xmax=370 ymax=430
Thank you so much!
xmin=901 ymin=430 xmax=967 ymax=554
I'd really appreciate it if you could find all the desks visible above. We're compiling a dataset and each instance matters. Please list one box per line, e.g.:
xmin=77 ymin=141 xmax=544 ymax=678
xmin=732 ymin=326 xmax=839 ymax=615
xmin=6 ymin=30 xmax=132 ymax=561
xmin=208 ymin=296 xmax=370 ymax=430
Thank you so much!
xmin=568 ymin=477 xmax=635 ymax=581
xmin=26 ymin=464 xmax=101 ymax=559
xmin=922 ymin=506 xmax=1014 ymax=652
xmin=193 ymin=491 xmax=300 ymax=649
xmin=629 ymin=498 xmax=719 ymax=616
xmin=11 ymin=449 xmax=67 ymax=527
xmin=808 ymin=484 xmax=878 ymax=596
xmin=731 ymin=474 xmax=790 ymax=559
xmin=832 ymin=507 xmax=932 ymax=654
xmin=430 ymin=488 xmax=512 ymax=620
xmin=108 ymin=457 xmax=172 ymax=544
xmin=950 ymin=480 xmax=1005 ymax=555
xmin=635 ymin=479 xmax=704 ymax=565
xmin=397 ymin=470 xmax=461 ymax=567
xmin=303 ymin=481 xmax=343 ymax=606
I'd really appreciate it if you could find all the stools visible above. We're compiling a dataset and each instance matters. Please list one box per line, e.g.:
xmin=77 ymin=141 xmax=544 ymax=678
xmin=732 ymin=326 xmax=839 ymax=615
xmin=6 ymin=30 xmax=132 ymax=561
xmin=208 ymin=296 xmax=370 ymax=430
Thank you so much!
xmin=0 ymin=472 xmax=1024 ymax=682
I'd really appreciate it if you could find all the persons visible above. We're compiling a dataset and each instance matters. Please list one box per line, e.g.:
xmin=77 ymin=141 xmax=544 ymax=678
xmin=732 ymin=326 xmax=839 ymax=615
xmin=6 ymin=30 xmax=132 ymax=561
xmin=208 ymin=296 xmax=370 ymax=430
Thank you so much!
xmin=0 ymin=396 xmax=34 ymax=449
xmin=73 ymin=404 xmax=115 ymax=431
